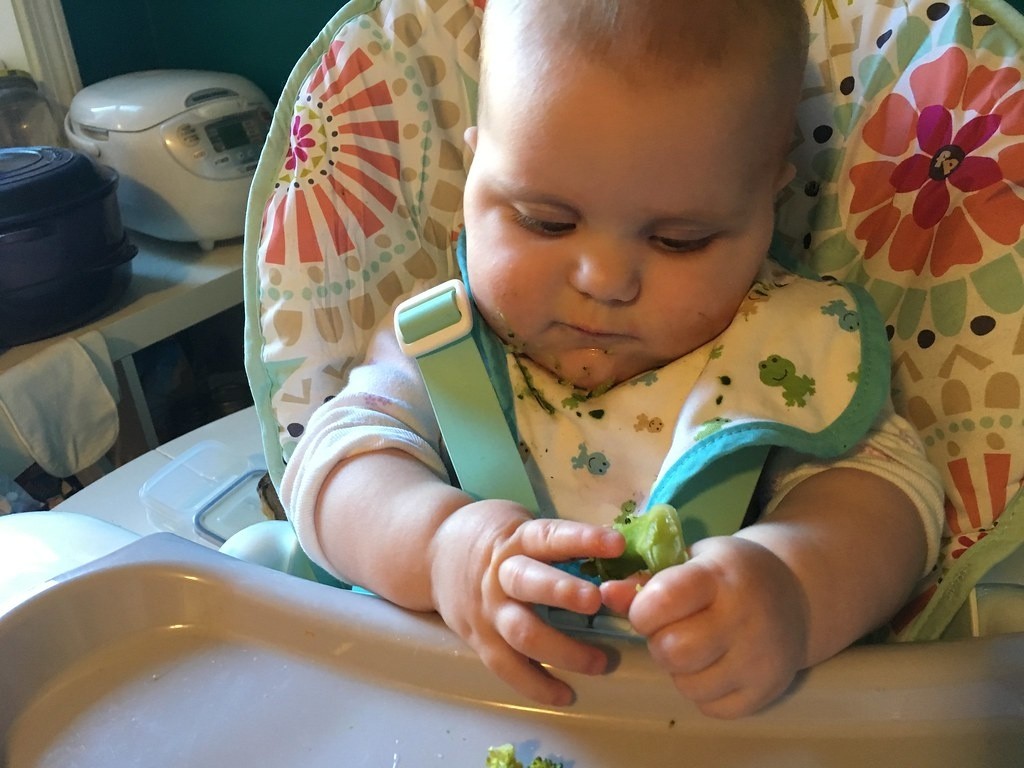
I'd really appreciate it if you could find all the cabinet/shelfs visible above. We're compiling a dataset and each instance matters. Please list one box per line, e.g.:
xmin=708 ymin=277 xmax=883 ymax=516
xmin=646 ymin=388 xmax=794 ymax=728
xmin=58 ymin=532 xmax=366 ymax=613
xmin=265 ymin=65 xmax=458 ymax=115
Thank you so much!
xmin=0 ymin=222 xmax=246 ymax=485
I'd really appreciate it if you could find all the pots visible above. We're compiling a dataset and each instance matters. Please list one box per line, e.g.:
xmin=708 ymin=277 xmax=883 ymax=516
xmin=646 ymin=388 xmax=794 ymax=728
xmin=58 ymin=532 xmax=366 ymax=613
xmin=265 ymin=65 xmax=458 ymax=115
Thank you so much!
xmin=0 ymin=146 xmax=139 ymax=349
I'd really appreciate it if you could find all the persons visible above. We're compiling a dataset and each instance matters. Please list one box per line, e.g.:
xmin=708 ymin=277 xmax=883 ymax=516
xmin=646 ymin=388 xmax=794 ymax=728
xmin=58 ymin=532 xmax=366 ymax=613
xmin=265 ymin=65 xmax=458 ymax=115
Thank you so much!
xmin=279 ymin=0 xmax=945 ymax=717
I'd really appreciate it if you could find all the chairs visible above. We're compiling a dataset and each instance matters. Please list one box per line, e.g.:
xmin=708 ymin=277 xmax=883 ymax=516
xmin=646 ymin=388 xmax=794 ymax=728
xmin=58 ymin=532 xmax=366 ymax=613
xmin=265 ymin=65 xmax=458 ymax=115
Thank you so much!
xmin=0 ymin=0 xmax=1024 ymax=768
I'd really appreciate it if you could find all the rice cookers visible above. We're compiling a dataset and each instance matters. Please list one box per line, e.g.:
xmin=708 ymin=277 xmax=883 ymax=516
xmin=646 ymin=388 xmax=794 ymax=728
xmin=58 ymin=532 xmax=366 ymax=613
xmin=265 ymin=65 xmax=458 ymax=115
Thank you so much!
xmin=64 ymin=69 xmax=277 ymax=252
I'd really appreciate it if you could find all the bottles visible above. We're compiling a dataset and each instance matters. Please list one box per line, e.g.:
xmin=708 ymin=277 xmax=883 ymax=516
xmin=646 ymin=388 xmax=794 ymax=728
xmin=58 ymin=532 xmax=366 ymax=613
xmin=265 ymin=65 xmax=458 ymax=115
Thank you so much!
xmin=0 ymin=68 xmax=64 ymax=148
